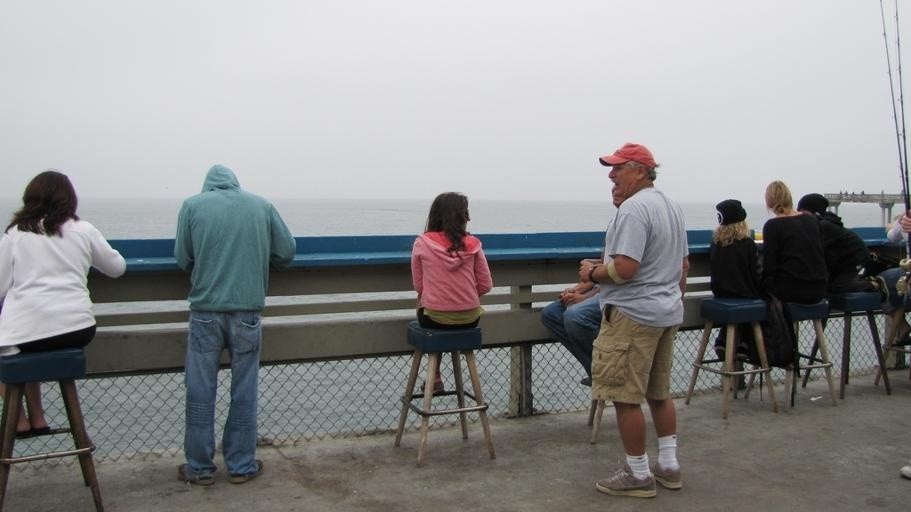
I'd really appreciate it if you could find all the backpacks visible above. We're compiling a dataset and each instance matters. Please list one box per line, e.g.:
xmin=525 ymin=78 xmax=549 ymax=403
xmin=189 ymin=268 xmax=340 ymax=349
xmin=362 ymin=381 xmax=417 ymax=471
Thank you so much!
xmin=749 ymin=289 xmax=797 ymax=369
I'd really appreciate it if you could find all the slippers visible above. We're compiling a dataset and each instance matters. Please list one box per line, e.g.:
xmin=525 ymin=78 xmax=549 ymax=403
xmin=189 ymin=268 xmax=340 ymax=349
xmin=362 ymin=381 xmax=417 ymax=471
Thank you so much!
xmin=32 ymin=427 xmax=51 ymax=437
xmin=892 ymin=335 xmax=910 ymax=345
xmin=15 ymin=428 xmax=32 ymax=438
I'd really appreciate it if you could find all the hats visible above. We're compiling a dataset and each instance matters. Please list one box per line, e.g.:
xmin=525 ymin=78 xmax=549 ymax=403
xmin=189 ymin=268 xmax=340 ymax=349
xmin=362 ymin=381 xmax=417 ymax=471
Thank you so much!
xmin=797 ymin=194 xmax=829 ymax=214
xmin=599 ymin=144 xmax=657 ymax=169
xmin=715 ymin=199 xmax=746 ymax=225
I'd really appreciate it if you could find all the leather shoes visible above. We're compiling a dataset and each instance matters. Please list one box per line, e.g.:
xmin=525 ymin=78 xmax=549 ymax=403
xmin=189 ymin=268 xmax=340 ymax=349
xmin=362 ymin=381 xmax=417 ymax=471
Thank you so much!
xmin=580 ymin=376 xmax=593 ymax=387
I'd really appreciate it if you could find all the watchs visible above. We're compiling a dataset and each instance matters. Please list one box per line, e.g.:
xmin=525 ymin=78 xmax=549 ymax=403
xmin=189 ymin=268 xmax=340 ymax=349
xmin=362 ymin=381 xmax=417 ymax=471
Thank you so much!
xmin=588 ymin=263 xmax=600 ymax=284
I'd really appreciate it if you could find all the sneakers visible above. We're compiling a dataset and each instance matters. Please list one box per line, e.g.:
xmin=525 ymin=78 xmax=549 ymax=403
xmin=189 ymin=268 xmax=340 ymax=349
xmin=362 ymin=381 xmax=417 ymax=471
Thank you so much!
xmin=864 ymin=276 xmax=876 ymax=281
xmin=649 ymin=462 xmax=683 ymax=489
xmin=714 ymin=338 xmax=726 ymax=359
xmin=596 ymin=469 xmax=656 ymax=498
xmin=875 ymin=276 xmax=889 ymax=301
xmin=421 ymin=374 xmax=444 ymax=393
xmin=178 ymin=463 xmax=215 ymax=485
xmin=736 ymin=343 xmax=747 ymax=358
xmin=228 ymin=460 xmax=263 ymax=484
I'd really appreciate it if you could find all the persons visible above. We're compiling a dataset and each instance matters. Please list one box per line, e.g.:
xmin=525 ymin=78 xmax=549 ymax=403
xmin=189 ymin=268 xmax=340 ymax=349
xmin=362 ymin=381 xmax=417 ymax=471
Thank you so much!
xmin=0 ymin=171 xmax=127 ymax=439
xmin=762 ymin=180 xmax=826 ymax=304
xmin=412 ymin=195 xmax=494 ymax=395
xmin=797 ymin=194 xmax=888 ymax=305
xmin=541 ymin=184 xmax=627 ymax=387
xmin=710 ymin=199 xmax=763 ymax=361
xmin=879 ymin=210 xmax=911 ymax=344
xmin=175 ymin=163 xmax=296 ymax=486
xmin=580 ymin=145 xmax=690 ymax=498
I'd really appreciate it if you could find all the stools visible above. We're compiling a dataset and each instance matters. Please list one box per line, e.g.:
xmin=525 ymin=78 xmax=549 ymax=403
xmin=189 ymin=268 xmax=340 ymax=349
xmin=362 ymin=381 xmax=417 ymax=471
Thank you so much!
xmin=875 ymin=305 xmax=910 ymax=386
xmin=685 ymin=298 xmax=780 ymax=418
xmin=394 ymin=322 xmax=496 ymax=466
xmin=0 ymin=348 xmax=104 ymax=512
xmin=744 ymin=299 xmax=838 ymax=413
xmin=587 ymin=391 xmax=608 ymax=444
xmin=804 ymin=293 xmax=893 ymax=398
